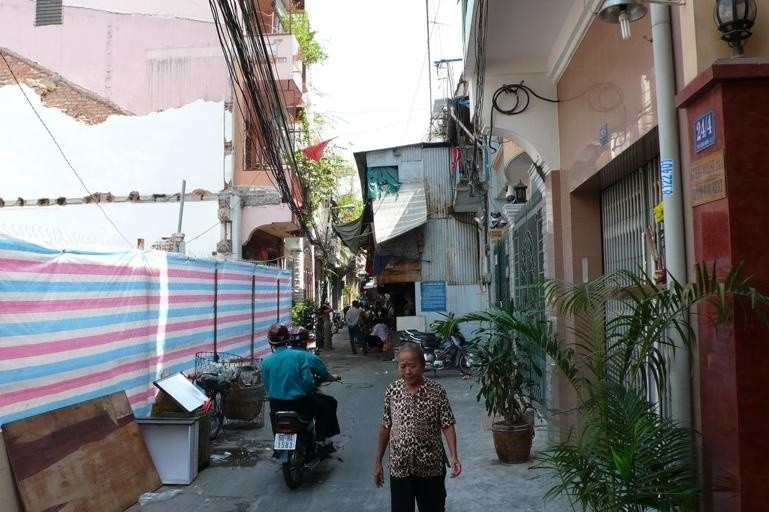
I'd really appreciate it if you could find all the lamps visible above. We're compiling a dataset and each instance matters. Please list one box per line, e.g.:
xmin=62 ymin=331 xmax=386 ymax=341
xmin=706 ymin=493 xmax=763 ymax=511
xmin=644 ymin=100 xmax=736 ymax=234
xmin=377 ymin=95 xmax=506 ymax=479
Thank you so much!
xmin=597 ymin=0 xmax=646 ymax=38
xmin=490 ymin=212 xmax=507 ymax=227
xmin=512 ymin=178 xmax=528 ymax=203
xmin=712 ymin=1 xmax=757 ymax=58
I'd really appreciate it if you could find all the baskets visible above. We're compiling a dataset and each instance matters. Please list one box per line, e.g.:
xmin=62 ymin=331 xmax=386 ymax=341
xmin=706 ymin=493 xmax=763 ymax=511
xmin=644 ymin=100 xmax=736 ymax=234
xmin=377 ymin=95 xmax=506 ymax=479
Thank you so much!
xmin=195 ymin=351 xmax=243 ymax=389
xmin=222 ymin=384 xmax=265 ymax=420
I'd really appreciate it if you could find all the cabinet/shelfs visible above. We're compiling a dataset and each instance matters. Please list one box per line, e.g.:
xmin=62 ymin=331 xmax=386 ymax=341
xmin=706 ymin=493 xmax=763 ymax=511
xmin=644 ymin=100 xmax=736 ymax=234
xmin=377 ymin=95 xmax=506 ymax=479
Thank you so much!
xmin=129 ymin=415 xmax=199 ymax=485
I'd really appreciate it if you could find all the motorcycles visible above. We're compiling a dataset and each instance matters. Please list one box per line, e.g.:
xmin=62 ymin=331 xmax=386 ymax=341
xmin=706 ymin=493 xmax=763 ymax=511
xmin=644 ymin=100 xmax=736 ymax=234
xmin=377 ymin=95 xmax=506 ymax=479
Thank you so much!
xmin=266 ymin=372 xmax=341 ymax=489
xmin=331 ymin=311 xmax=342 ymax=334
xmin=390 ymin=313 xmax=486 ymax=379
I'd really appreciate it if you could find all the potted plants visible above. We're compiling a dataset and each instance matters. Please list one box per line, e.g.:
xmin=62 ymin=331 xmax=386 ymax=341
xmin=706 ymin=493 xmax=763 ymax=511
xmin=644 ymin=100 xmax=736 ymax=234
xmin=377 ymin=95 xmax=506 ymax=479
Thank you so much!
xmin=429 ymin=293 xmax=546 ymax=462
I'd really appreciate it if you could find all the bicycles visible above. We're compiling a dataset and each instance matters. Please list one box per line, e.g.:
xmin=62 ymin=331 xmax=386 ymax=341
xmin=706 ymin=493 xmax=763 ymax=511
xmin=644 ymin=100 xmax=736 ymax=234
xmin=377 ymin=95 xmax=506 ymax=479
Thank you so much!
xmin=188 ymin=352 xmax=240 ymax=444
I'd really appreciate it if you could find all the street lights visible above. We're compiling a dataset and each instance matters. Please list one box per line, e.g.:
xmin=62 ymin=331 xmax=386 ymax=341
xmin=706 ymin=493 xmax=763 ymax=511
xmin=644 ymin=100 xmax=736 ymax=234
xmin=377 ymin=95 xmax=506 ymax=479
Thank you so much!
xmin=322 ymin=202 xmax=355 ymax=310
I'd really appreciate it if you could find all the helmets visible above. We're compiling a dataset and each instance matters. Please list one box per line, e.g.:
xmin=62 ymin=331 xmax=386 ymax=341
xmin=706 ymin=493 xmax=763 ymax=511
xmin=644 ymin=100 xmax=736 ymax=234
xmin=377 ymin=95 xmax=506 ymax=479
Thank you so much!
xmin=267 ymin=324 xmax=289 ymax=344
xmin=289 ymin=326 xmax=308 ymax=347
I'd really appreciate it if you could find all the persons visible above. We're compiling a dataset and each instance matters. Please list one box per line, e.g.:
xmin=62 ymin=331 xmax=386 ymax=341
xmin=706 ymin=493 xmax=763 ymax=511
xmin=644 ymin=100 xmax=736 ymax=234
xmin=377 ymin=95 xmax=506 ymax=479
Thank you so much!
xmin=287 ymin=325 xmax=343 ymax=447
xmin=260 ymin=322 xmax=338 ymax=447
xmin=373 ymin=340 xmax=463 ymax=511
xmin=310 ymin=286 xmax=412 ymax=359
